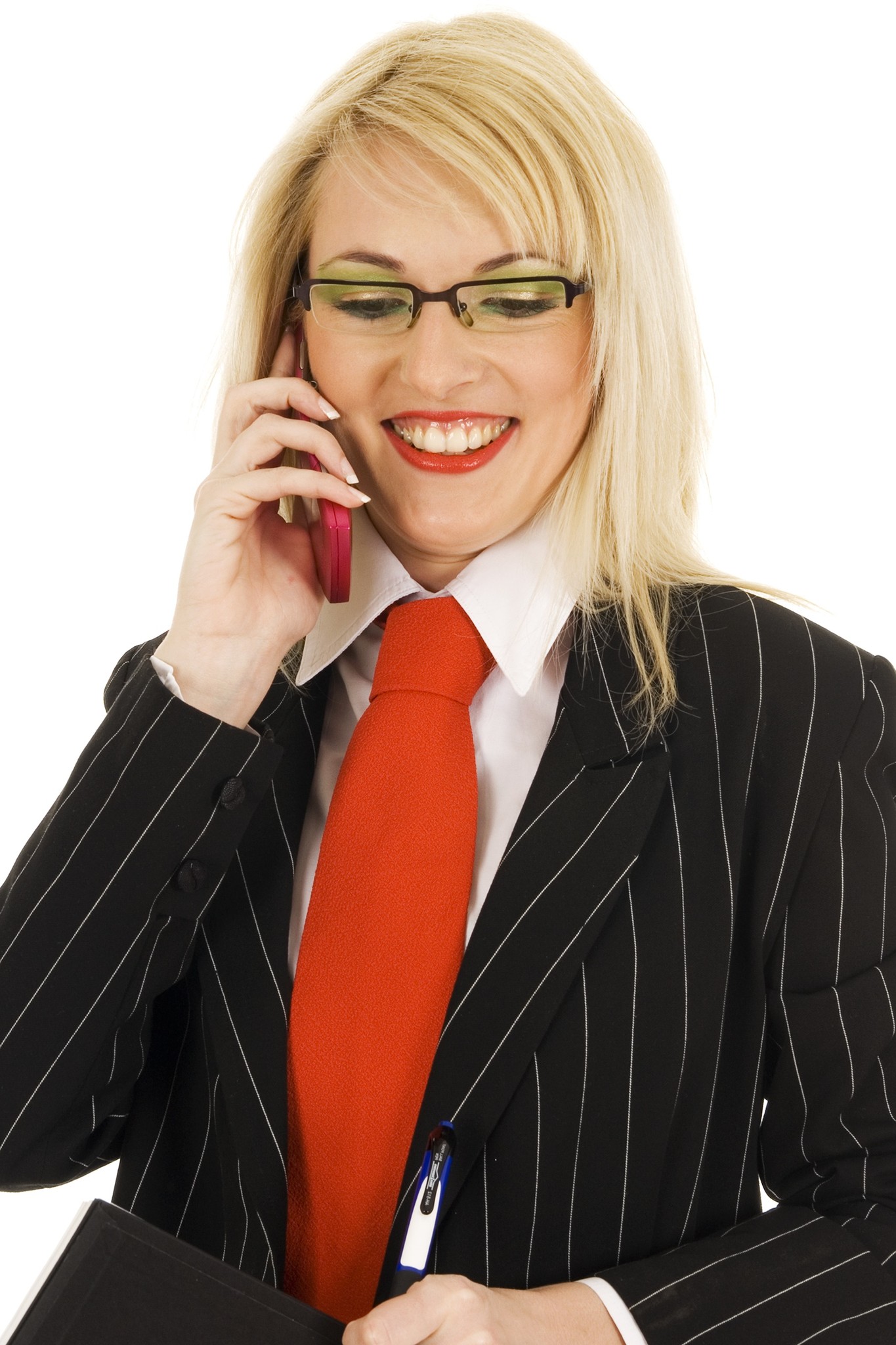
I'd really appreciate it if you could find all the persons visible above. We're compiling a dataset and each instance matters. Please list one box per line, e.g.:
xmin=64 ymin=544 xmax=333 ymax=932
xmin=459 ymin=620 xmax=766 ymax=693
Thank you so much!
xmin=1 ymin=7 xmax=896 ymax=1345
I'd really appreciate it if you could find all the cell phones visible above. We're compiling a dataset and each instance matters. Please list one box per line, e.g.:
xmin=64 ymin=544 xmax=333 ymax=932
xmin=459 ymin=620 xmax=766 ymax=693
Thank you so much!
xmin=289 ymin=334 xmax=353 ymax=603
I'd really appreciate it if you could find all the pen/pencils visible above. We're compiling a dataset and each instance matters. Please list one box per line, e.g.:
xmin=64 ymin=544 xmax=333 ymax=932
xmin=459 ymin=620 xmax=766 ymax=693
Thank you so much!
xmin=393 ymin=1121 xmax=455 ymax=1297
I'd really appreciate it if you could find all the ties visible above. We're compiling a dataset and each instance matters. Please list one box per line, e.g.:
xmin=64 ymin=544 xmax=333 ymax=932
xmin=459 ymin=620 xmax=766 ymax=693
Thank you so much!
xmin=288 ymin=595 xmax=498 ymax=1325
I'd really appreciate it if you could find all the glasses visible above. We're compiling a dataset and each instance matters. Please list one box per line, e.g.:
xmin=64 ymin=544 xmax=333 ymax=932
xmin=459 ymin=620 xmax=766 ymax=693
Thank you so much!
xmin=292 ymin=269 xmax=592 ymax=336
